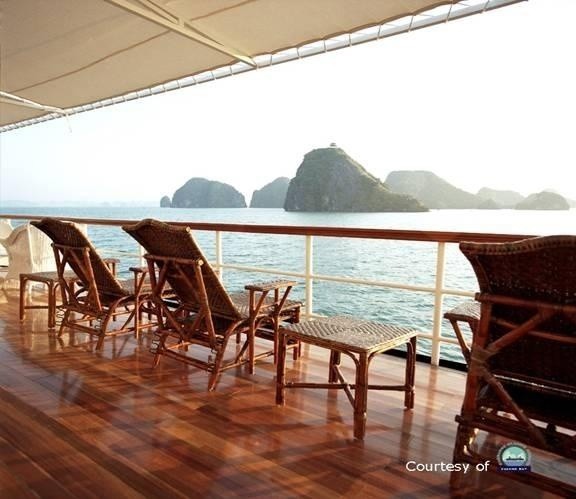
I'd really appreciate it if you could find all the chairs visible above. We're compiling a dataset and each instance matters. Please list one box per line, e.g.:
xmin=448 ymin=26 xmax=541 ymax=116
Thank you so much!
xmin=122 ymin=220 xmax=303 ymax=397
xmin=438 ymin=228 xmax=575 ymax=499
xmin=30 ymin=216 xmax=178 ymax=350
xmin=0 ymin=217 xmax=80 ymax=327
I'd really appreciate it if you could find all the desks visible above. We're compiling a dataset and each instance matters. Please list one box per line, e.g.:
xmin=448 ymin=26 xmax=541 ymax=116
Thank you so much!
xmin=277 ymin=313 xmax=420 ymax=438
